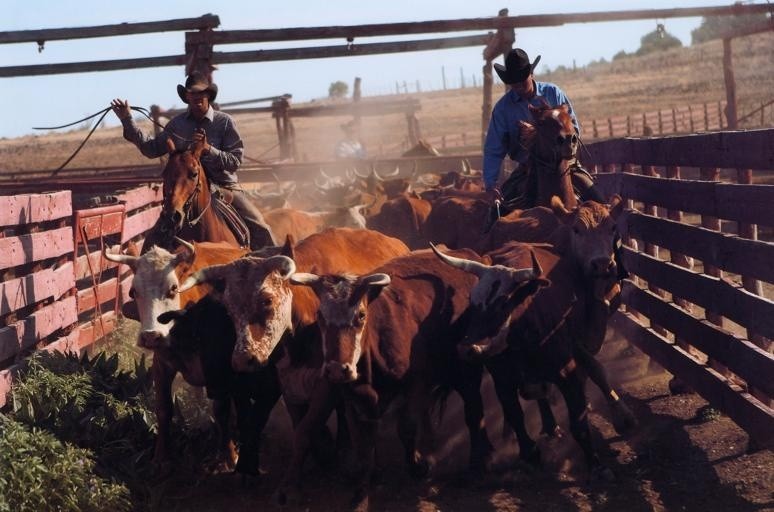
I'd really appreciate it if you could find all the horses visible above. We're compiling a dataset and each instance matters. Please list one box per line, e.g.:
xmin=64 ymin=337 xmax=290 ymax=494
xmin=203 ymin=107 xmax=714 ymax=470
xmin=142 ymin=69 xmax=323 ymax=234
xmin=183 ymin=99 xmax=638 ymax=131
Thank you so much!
xmin=159 ymin=133 xmax=241 ymax=249
xmin=501 ymin=103 xmax=583 ymax=213
xmin=400 ymin=139 xmax=442 ymax=157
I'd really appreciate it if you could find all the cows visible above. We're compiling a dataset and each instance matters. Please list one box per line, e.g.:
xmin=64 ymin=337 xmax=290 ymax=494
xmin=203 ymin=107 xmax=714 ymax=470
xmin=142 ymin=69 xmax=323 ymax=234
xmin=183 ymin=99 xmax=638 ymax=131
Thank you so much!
xmin=429 ymin=241 xmax=637 ymax=470
xmin=288 ymin=248 xmax=495 ymax=457
xmin=489 ymin=193 xmax=624 ymax=279
xmin=176 ymin=226 xmax=411 ymax=472
xmin=241 ymin=156 xmax=492 ymax=255
xmin=102 ymin=236 xmax=252 ymax=469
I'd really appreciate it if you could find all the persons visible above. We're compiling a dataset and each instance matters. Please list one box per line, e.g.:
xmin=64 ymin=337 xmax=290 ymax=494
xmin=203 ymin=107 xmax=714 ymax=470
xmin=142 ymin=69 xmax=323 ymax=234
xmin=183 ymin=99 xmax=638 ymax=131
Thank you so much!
xmin=481 ymin=48 xmax=605 ymax=210
xmin=336 ymin=120 xmax=366 ymax=159
xmin=110 ymin=71 xmax=276 ymax=253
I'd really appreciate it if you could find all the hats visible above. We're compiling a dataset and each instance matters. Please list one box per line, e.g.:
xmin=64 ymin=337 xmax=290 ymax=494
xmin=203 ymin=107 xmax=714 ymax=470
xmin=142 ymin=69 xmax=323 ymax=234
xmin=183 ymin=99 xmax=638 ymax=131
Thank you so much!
xmin=493 ymin=48 xmax=541 ymax=85
xmin=174 ymin=70 xmax=218 ymax=104
